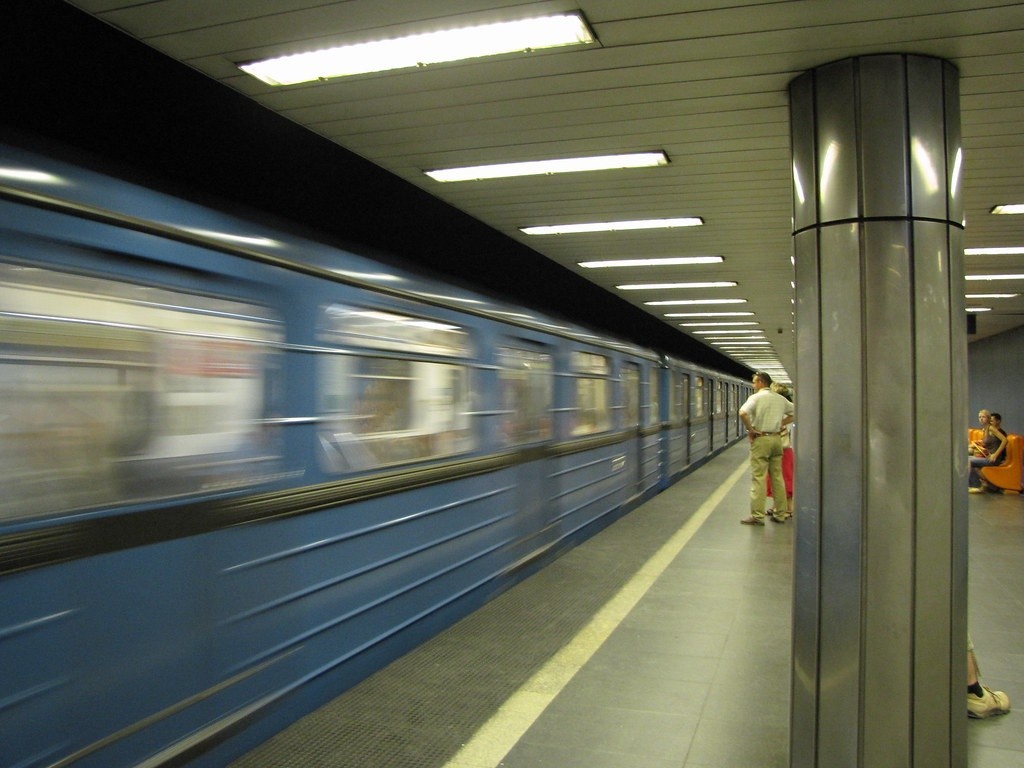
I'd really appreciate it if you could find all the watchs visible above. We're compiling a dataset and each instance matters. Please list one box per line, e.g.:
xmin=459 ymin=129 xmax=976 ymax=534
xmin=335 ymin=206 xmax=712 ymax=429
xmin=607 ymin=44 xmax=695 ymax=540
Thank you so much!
xmin=746 ymin=427 xmax=753 ymax=433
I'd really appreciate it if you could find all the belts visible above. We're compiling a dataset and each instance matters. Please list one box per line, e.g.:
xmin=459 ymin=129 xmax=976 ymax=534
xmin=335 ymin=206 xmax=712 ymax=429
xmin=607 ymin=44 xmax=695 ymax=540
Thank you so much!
xmin=754 ymin=432 xmax=781 ymax=437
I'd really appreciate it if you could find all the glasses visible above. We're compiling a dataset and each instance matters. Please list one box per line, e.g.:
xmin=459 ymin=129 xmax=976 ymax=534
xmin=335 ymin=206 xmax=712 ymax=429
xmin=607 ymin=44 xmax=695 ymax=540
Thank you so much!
xmin=756 ymin=371 xmax=761 ymax=376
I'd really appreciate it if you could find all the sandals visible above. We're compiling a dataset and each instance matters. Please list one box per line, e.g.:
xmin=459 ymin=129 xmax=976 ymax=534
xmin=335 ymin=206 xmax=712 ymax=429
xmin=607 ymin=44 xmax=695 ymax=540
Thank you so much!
xmin=784 ymin=511 xmax=793 ymax=519
xmin=765 ymin=508 xmax=773 ymax=515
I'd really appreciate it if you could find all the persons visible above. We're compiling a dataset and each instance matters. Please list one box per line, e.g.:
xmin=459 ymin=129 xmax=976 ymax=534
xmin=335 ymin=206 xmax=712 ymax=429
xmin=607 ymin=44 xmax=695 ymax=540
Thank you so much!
xmin=739 ymin=371 xmax=795 ymax=526
xmin=968 ymin=409 xmax=1008 ymax=494
xmin=967 ymin=634 xmax=1010 ymax=718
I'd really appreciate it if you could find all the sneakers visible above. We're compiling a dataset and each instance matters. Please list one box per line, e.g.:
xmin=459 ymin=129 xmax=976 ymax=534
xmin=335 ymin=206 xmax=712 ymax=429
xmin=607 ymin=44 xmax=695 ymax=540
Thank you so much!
xmin=968 ymin=685 xmax=1011 ymax=719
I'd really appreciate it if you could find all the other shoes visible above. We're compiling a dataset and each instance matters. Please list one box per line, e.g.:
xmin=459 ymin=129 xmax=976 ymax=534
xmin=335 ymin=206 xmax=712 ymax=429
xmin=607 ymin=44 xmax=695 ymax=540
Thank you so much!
xmin=968 ymin=486 xmax=984 ymax=493
xmin=740 ymin=515 xmax=764 ymax=526
xmin=770 ymin=516 xmax=784 ymax=523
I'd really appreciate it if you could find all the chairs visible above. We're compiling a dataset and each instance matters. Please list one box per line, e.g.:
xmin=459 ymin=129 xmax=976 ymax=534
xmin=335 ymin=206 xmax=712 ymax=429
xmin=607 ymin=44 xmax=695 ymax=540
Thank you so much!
xmin=972 ymin=429 xmax=981 ymax=444
xmin=978 ymin=433 xmax=1024 ymax=495
xmin=978 ymin=431 xmax=984 ymax=441
xmin=969 ymin=428 xmax=974 ymax=444
